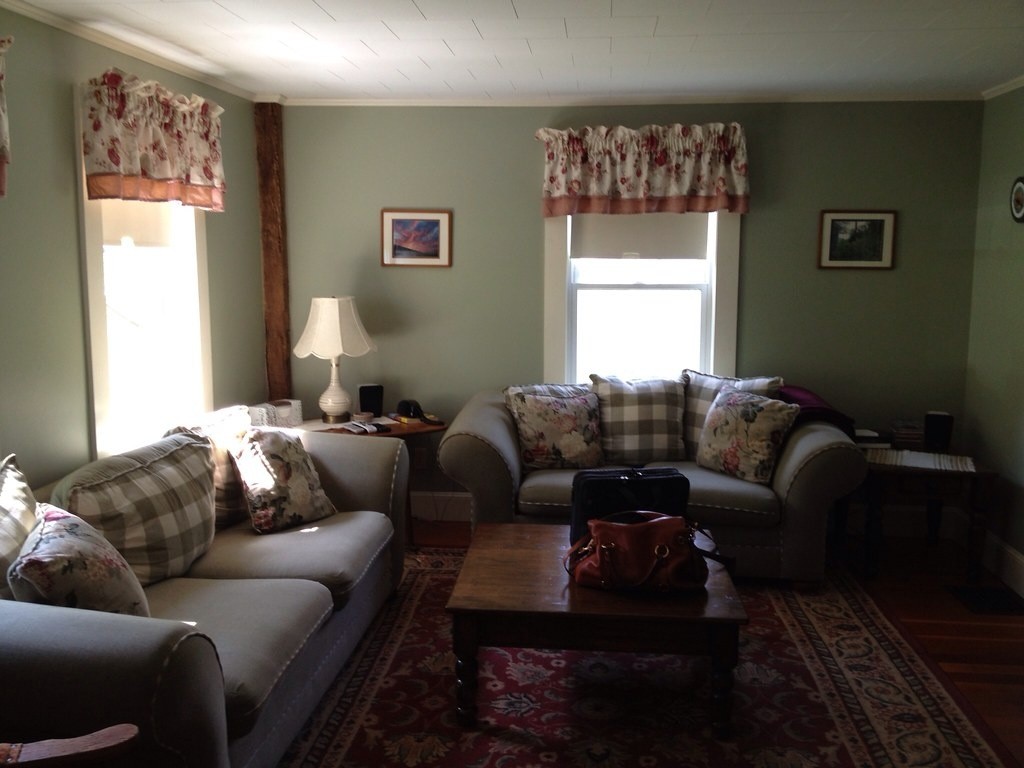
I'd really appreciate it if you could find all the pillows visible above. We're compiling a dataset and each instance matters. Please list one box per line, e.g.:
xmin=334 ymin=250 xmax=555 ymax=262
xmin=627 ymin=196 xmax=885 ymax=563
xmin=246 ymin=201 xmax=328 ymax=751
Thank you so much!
xmin=696 ymin=384 xmax=800 ymax=486
xmin=682 ymin=369 xmax=784 ymax=460
xmin=228 ymin=426 xmax=338 ymax=533
xmin=56 ymin=436 xmax=216 ymax=588
xmin=207 ymin=406 xmax=251 ymax=534
xmin=502 ymin=384 xmax=605 ymax=473
xmin=0 ymin=452 xmax=36 ymax=601
xmin=588 ymin=374 xmax=690 ymax=462
xmin=6 ymin=502 xmax=150 ymax=619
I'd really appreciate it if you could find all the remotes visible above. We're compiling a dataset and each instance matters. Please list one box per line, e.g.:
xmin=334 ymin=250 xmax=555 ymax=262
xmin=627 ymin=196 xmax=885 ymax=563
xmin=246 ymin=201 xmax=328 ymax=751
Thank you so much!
xmin=344 ymin=420 xmax=391 ymax=435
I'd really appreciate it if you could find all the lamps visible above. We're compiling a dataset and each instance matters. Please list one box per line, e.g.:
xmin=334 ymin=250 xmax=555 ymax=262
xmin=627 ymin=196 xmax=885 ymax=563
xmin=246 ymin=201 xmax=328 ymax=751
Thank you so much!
xmin=293 ymin=296 xmax=377 ymax=424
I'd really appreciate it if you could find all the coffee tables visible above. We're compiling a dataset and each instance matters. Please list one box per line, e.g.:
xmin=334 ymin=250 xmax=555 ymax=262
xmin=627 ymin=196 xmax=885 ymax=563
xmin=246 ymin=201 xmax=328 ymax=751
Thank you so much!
xmin=445 ymin=522 xmax=749 ymax=739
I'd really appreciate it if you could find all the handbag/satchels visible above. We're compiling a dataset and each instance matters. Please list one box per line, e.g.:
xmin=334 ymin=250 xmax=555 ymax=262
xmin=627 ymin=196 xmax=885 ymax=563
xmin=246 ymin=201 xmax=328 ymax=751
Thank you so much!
xmin=564 ymin=511 xmax=709 ymax=591
xmin=570 ymin=467 xmax=690 ymax=545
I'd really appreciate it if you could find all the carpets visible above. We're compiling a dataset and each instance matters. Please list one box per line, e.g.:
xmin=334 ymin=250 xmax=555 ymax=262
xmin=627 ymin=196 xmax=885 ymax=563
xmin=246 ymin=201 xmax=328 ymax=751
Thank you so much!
xmin=272 ymin=543 xmax=1022 ymax=768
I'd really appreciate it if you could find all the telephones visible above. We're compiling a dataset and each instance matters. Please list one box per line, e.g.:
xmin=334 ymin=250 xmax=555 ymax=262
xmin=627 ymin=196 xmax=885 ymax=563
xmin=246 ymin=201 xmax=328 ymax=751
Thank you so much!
xmin=397 ymin=399 xmax=422 ymax=417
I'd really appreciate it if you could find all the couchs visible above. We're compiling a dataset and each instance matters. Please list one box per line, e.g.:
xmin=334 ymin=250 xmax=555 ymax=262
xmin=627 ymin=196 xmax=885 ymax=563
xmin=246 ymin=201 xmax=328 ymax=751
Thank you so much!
xmin=0 ymin=427 xmax=409 ymax=768
xmin=436 ymin=392 xmax=856 ymax=522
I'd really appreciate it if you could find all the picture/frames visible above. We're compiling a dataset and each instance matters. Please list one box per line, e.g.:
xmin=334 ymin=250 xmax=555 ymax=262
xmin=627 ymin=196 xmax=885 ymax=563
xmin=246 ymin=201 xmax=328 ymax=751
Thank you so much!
xmin=1009 ymin=176 xmax=1024 ymax=223
xmin=815 ymin=209 xmax=899 ymax=271
xmin=380 ymin=208 xmax=452 ymax=267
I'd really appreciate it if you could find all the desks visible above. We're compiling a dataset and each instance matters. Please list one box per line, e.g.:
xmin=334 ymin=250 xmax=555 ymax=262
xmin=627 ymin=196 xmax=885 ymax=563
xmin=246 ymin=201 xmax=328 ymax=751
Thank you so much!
xmin=829 ymin=448 xmax=999 ymax=585
xmin=303 ymin=414 xmax=449 ymax=491
